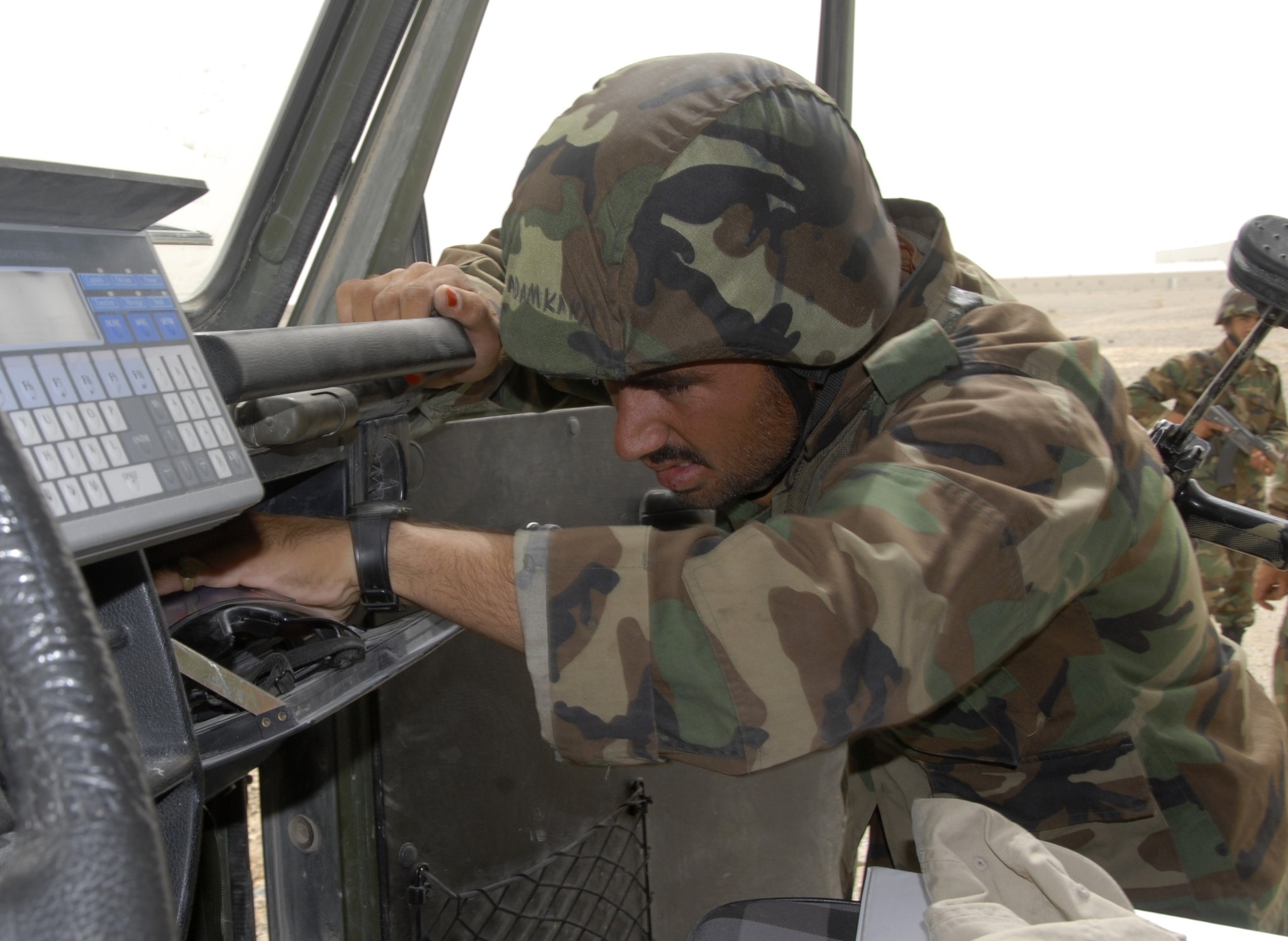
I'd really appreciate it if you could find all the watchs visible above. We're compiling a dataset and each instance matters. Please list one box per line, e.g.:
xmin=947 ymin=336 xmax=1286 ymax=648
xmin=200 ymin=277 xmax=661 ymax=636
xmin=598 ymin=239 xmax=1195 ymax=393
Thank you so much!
xmin=345 ymin=498 xmax=413 ymax=614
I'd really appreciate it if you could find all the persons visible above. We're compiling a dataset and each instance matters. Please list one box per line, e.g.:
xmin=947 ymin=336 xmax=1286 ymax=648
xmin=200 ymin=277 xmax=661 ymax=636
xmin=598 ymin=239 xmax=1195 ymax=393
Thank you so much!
xmin=150 ymin=52 xmax=1288 ymax=937
xmin=1252 ymin=444 xmax=1288 ymax=725
xmin=1126 ymin=289 xmax=1288 ymax=647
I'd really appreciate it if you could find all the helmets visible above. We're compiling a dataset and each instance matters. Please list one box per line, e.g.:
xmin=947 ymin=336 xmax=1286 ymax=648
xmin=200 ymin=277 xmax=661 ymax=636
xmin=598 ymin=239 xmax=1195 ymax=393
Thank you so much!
xmin=499 ymin=53 xmax=901 ymax=383
xmin=1214 ymin=287 xmax=1258 ymax=325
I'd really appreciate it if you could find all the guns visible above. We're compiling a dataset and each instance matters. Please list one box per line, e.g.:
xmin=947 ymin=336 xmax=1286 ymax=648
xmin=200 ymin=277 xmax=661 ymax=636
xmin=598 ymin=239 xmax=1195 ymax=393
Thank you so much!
xmin=1145 ymin=307 xmax=1287 ymax=572
xmin=1201 ymin=403 xmax=1285 ymax=491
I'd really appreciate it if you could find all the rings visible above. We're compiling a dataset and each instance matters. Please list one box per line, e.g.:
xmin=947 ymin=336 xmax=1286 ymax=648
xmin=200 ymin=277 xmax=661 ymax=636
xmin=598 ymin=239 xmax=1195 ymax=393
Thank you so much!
xmin=181 ymin=574 xmax=196 ymax=591
